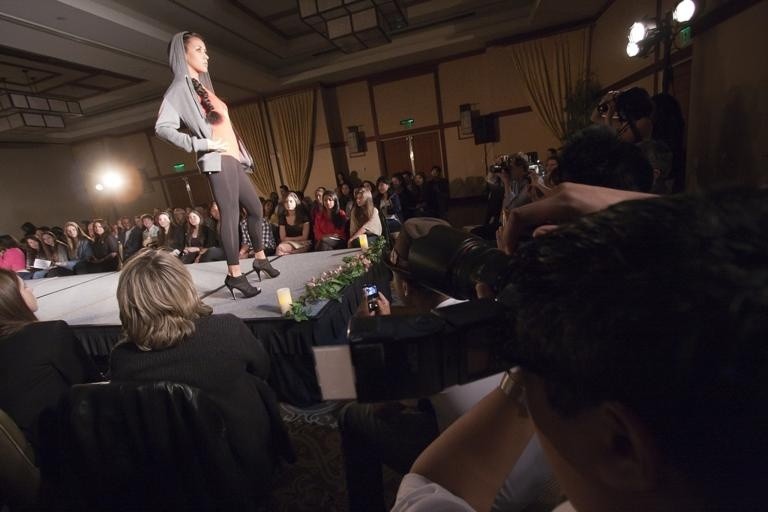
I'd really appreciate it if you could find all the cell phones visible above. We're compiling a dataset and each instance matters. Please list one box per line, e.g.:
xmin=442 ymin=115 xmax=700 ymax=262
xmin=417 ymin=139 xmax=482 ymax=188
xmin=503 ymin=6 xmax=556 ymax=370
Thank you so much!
xmin=362 ymin=282 xmax=380 ymax=312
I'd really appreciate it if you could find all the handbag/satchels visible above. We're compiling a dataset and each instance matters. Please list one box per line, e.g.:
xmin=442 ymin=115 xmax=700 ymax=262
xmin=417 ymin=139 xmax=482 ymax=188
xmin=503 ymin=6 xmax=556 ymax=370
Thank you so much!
xmin=381 ymin=200 xmax=402 ymax=234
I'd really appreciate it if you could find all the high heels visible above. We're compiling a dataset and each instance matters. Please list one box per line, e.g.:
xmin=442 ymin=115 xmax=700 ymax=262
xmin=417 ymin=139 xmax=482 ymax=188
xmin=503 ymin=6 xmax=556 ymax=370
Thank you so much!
xmin=225 ymin=272 xmax=262 ymax=300
xmin=252 ymin=257 xmax=282 ymax=282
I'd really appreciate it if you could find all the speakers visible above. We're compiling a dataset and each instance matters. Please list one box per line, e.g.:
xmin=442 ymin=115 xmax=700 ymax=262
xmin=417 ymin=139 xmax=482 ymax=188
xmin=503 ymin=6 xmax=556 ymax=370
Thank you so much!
xmin=471 ymin=115 xmax=500 ymax=144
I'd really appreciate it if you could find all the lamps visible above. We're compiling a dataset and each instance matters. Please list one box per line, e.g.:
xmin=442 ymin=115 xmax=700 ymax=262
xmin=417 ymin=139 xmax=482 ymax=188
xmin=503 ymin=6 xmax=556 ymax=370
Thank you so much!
xmin=276 ymin=287 xmax=292 ymax=315
xmin=673 ymin=0 xmax=697 ymax=24
xmin=359 ymin=233 xmax=370 ymax=251
xmin=625 ymin=16 xmax=664 ymax=58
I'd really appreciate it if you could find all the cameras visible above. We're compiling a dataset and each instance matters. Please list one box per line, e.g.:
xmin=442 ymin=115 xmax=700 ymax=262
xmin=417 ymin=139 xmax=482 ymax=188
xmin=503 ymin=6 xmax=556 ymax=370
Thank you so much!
xmin=598 ymin=104 xmax=608 ymax=113
xmin=383 ymin=219 xmax=532 ymax=366
xmin=490 ymin=162 xmax=510 ymax=173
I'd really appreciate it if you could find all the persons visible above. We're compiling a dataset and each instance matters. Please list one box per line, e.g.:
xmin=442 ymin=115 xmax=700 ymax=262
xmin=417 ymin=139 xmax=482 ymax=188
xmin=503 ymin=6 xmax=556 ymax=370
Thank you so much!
xmin=155 ymin=30 xmax=280 ymax=301
xmin=0 ymin=117 xmax=767 ymax=511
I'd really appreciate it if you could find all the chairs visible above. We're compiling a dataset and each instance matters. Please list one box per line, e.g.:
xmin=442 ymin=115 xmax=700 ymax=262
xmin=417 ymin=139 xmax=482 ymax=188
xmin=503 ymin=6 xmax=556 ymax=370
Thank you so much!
xmin=68 ymin=380 xmax=246 ymax=510
xmin=338 ymin=299 xmax=525 ymax=512
xmin=347 ymin=210 xmax=390 ymax=251
xmin=0 ymin=410 xmax=40 ymax=512
